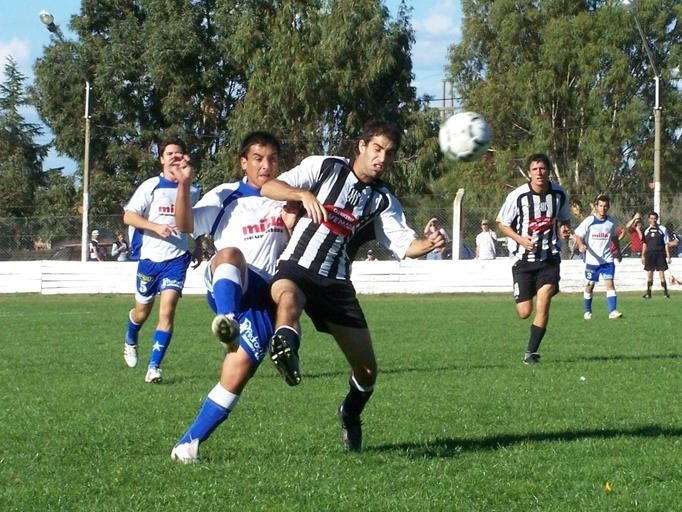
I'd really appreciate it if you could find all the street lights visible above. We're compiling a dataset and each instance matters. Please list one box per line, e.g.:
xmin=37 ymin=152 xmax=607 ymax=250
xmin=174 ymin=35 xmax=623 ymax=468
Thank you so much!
xmin=39 ymin=11 xmax=93 ymax=261
xmin=619 ymin=1 xmax=661 ymax=227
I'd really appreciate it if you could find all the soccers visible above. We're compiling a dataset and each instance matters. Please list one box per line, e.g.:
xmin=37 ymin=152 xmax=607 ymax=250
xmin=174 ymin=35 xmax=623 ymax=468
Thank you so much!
xmin=437 ymin=112 xmax=494 ymax=162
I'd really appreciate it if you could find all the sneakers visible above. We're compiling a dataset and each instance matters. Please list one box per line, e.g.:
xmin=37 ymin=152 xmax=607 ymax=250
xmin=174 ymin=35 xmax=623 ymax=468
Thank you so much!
xmin=211 ymin=314 xmax=240 ymax=353
xmin=269 ymin=335 xmax=301 ymax=386
xmin=124 ymin=342 xmax=138 ymax=367
xmin=171 ymin=439 xmax=200 ymax=466
xmin=609 ymin=309 xmax=623 ymax=319
xmin=338 ymin=401 xmax=361 ymax=453
xmin=523 ymin=352 xmax=540 ymax=369
xmin=144 ymin=369 xmax=161 ymax=384
xmin=584 ymin=311 xmax=592 ymax=320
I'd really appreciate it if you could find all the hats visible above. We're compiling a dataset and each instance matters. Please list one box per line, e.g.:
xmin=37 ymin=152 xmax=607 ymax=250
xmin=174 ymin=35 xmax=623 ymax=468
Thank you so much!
xmin=91 ymin=230 xmax=99 ymax=236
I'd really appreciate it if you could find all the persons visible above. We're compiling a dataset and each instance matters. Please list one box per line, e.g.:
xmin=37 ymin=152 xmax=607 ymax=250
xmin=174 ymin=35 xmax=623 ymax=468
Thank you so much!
xmin=475 ymin=219 xmax=498 ymax=259
xmin=167 ymin=132 xmax=302 ymax=463
xmin=89 ymin=230 xmax=102 ymax=261
xmin=423 ymin=218 xmax=450 ymax=260
xmin=496 ymin=153 xmax=572 ymax=366
xmin=364 ymin=249 xmax=379 ymax=261
xmin=259 ymin=119 xmax=447 ymax=452
xmin=122 ymin=137 xmax=202 ymax=383
xmin=574 ymin=195 xmax=682 ymax=320
xmin=110 ymin=232 xmax=129 ymax=262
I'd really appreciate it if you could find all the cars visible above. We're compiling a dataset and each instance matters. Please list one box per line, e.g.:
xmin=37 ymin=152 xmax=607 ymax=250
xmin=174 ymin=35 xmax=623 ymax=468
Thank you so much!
xmin=621 ymin=235 xmax=681 ymax=257
xmin=495 ymin=236 xmax=510 ymax=258
xmin=48 ymin=240 xmax=115 ymax=261
